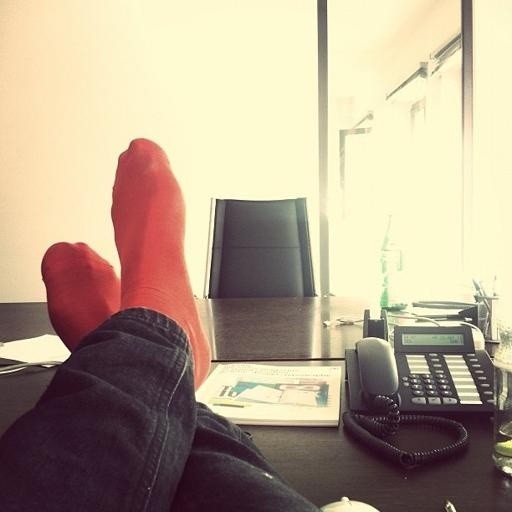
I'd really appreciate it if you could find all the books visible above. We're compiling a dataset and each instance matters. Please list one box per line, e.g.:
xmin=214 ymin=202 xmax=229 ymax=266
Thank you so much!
xmin=190 ymin=360 xmax=345 ymax=429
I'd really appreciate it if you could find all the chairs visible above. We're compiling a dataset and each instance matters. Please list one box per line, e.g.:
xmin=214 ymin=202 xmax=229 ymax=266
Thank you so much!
xmin=203 ymin=197 xmax=319 ymax=297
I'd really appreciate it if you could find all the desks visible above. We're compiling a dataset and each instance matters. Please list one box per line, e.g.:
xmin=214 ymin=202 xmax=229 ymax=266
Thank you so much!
xmin=1 ymin=296 xmax=511 ymax=512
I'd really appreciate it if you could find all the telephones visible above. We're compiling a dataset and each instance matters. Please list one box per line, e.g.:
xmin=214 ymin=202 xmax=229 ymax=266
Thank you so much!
xmin=341 ymin=325 xmax=505 ymax=470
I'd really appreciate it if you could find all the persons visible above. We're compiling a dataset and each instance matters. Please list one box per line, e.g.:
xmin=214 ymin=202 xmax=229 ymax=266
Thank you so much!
xmin=0 ymin=135 xmax=320 ymax=512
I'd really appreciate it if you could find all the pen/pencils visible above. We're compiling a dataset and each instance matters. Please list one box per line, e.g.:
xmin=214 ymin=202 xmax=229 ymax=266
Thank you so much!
xmin=472 ymin=275 xmax=498 ymax=340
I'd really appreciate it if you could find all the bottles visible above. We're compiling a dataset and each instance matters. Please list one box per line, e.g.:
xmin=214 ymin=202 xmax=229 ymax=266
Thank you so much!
xmin=380 ymin=213 xmax=411 ymax=311
xmin=493 ymin=321 xmax=512 ymax=475
